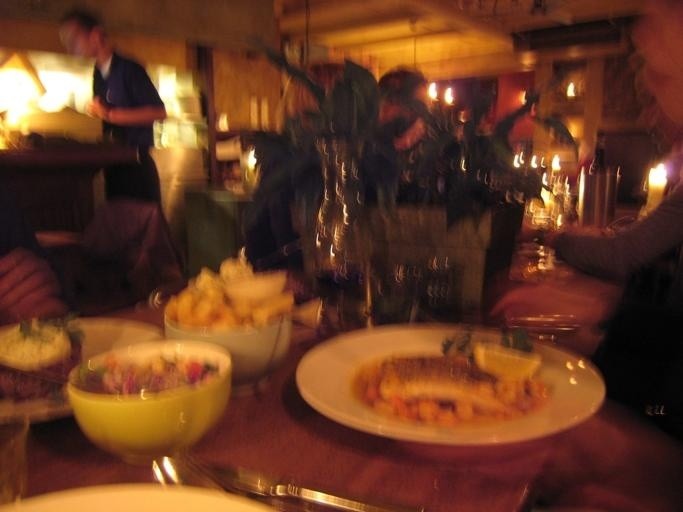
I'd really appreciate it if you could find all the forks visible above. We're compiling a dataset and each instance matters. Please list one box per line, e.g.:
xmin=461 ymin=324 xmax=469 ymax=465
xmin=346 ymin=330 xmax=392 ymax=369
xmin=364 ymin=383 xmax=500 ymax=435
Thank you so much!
xmin=182 ymin=451 xmax=375 ymax=511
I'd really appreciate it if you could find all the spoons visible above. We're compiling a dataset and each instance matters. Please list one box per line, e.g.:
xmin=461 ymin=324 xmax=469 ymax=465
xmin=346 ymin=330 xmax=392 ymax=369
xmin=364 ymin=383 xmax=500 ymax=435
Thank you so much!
xmin=148 ymin=455 xmax=224 ymax=489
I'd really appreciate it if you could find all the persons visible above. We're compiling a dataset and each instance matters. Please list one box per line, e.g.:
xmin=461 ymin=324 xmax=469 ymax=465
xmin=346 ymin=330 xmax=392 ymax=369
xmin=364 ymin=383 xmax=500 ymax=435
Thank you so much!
xmin=0 ymin=184 xmax=71 ymax=326
xmin=515 ymin=180 xmax=683 ymax=413
xmin=52 ymin=7 xmax=166 ymax=208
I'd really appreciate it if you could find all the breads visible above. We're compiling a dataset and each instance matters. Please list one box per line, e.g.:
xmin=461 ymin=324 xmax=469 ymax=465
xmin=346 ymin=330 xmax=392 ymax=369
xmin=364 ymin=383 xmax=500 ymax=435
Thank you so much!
xmin=380 ymin=357 xmax=507 ymax=412
xmin=164 ymin=256 xmax=294 ymax=330
xmin=0 ymin=316 xmax=73 ymax=372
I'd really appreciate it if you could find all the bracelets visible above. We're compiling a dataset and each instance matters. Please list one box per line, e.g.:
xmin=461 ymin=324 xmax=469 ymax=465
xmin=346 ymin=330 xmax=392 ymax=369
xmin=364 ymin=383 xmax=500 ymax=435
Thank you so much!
xmin=532 ymin=229 xmax=547 ymax=245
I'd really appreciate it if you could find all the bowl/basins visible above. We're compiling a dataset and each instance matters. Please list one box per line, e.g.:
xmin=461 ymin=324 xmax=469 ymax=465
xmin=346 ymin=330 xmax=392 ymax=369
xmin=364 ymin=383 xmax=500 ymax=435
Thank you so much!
xmin=161 ymin=300 xmax=290 ymax=396
xmin=70 ymin=339 xmax=234 ymax=466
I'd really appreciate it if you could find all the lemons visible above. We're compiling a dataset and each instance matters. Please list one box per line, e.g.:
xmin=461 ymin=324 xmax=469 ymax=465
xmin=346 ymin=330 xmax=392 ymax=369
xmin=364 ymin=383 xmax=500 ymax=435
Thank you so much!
xmin=474 ymin=340 xmax=542 ymax=383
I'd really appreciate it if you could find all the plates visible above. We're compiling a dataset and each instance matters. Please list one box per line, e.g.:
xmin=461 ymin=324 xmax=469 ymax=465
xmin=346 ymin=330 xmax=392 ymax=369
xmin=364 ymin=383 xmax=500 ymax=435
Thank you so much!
xmin=291 ymin=323 xmax=606 ymax=462
xmin=0 ymin=319 xmax=161 ymax=421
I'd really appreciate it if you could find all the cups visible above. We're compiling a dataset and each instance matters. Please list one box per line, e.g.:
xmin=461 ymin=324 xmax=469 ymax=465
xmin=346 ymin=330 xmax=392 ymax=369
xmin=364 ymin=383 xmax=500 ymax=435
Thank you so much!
xmin=576 ymin=166 xmax=622 ymax=227
